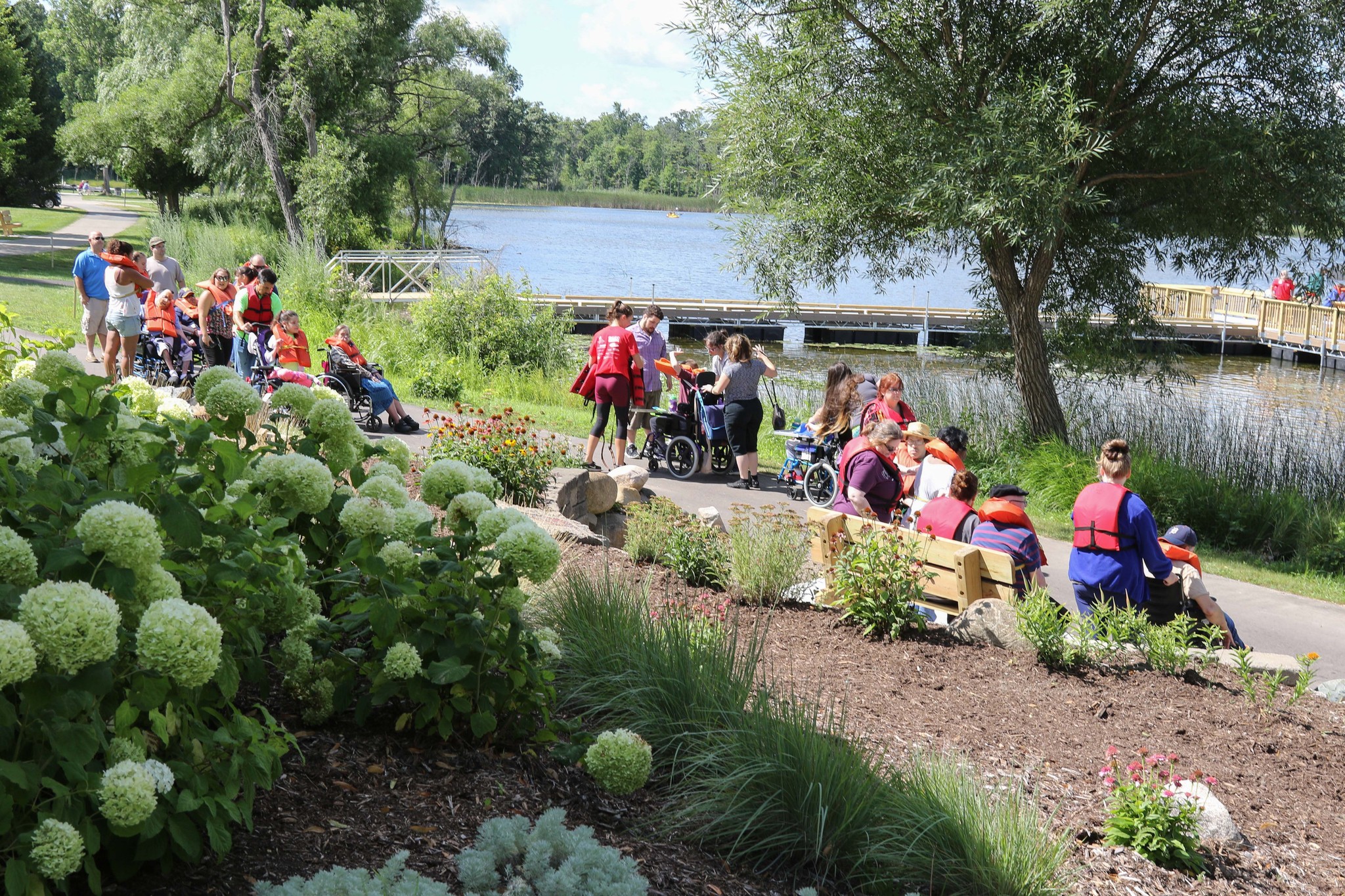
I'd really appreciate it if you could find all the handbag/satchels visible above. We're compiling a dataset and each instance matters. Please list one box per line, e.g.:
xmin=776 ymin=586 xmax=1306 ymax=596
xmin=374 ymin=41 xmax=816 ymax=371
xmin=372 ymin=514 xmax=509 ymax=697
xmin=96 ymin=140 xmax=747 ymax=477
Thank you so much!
xmin=698 ymin=447 xmax=712 ymax=474
xmin=772 ymin=402 xmax=786 ymax=431
xmin=701 ymin=403 xmax=728 ymax=440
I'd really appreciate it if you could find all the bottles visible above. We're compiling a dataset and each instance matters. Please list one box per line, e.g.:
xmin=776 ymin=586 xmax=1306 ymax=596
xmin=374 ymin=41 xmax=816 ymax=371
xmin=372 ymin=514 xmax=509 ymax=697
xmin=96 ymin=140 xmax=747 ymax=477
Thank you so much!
xmin=669 ymin=393 xmax=677 ymax=413
xmin=792 ymin=416 xmax=801 ymax=430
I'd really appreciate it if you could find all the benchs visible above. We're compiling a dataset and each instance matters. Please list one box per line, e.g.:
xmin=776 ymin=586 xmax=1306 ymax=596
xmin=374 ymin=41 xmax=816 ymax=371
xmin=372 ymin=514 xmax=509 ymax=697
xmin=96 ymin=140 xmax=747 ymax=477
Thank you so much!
xmin=807 ymin=505 xmax=1018 ymax=618
xmin=0 ymin=210 xmax=22 ymax=235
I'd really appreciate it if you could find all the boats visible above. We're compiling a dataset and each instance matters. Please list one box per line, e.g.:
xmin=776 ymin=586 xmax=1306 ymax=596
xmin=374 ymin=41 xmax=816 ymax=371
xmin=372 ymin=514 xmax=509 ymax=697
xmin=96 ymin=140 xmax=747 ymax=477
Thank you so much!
xmin=666 ymin=211 xmax=680 ymax=218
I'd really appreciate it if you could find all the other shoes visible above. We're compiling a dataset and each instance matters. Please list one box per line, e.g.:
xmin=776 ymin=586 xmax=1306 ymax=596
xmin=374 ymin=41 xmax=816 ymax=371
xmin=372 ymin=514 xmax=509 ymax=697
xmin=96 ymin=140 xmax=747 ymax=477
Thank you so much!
xmin=402 ymin=415 xmax=419 ymax=431
xmin=784 ymin=470 xmax=814 ymax=484
xmin=726 ymin=480 xmax=749 ymax=490
xmin=644 ymin=448 xmax=667 ymax=460
xmin=168 ymin=376 xmax=192 ymax=387
xmin=86 ymin=354 xmax=119 ymax=363
xmin=749 ymin=478 xmax=759 ymax=488
xmin=394 ymin=418 xmax=411 ymax=433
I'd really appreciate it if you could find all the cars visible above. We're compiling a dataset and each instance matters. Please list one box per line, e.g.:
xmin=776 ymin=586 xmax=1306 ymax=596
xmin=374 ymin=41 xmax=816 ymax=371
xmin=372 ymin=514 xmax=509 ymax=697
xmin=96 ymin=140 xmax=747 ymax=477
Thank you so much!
xmin=0 ymin=176 xmax=62 ymax=209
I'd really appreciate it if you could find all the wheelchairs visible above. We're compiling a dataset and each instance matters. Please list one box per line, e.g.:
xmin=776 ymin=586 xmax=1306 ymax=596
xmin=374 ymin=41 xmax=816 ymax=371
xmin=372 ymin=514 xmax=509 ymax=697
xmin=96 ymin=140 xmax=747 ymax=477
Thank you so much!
xmin=245 ymin=322 xmax=292 ymax=422
xmin=314 ymin=344 xmax=396 ymax=433
xmin=640 ymin=371 xmax=735 ymax=480
xmin=772 ymin=423 xmax=853 ymax=508
xmin=115 ymin=315 xmax=208 ymax=399
xmin=1127 ymin=573 xmax=1254 ymax=652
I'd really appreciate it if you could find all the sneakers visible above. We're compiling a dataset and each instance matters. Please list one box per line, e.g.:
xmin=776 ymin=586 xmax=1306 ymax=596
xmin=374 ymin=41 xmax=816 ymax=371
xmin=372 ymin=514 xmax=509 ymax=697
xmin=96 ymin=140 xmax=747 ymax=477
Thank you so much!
xmin=644 ymin=441 xmax=650 ymax=453
xmin=624 ymin=444 xmax=640 ymax=459
xmin=582 ymin=461 xmax=601 ymax=471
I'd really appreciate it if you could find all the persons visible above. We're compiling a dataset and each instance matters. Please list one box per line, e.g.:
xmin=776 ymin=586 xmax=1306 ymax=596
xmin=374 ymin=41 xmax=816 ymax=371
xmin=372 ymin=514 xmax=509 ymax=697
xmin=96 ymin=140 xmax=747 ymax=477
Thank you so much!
xmin=857 ymin=373 xmax=918 ymax=435
xmin=1069 ymin=440 xmax=1180 ymax=642
xmin=902 ymin=426 xmax=969 ymax=533
xmin=887 ymin=421 xmax=937 ymax=528
xmin=916 ymin=470 xmax=980 ymax=544
xmin=1270 ymin=269 xmax=1345 ymax=307
xmin=72 ymin=231 xmax=421 ymax=434
xmin=570 ymin=300 xmax=645 ymax=473
xmin=77 ymin=179 xmax=93 ymax=196
xmin=829 ymin=418 xmax=903 ymax=525
xmin=625 ymin=305 xmax=673 ymax=462
xmin=650 ymin=328 xmax=916 ymax=491
xmin=1141 ymin=524 xmax=1253 ymax=656
xmin=969 ymin=485 xmax=1072 ymax=635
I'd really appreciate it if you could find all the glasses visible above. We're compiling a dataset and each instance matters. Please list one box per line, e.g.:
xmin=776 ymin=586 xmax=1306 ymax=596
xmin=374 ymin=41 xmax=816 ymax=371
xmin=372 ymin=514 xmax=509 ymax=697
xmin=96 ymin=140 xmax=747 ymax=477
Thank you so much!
xmin=888 ymin=387 xmax=904 ymax=392
xmin=214 ymin=275 xmax=229 ymax=282
xmin=250 ymin=262 xmax=265 ymax=270
xmin=705 ymin=345 xmax=718 ymax=352
xmin=92 ymin=237 xmax=105 ymax=241
xmin=906 ymin=439 xmax=927 ymax=446
xmin=885 ymin=444 xmax=899 ymax=452
xmin=1010 ymin=499 xmax=1027 ymax=507
xmin=185 ymin=294 xmax=195 ymax=299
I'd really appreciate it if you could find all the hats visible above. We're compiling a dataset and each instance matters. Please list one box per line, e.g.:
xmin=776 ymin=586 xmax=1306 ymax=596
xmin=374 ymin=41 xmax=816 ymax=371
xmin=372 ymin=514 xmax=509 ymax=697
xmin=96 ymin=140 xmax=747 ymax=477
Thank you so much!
xmin=178 ymin=286 xmax=195 ymax=298
xmin=901 ymin=421 xmax=935 ymax=442
xmin=1157 ymin=525 xmax=1197 ymax=547
xmin=149 ymin=236 xmax=166 ymax=247
xmin=990 ymin=484 xmax=1029 ymax=498
